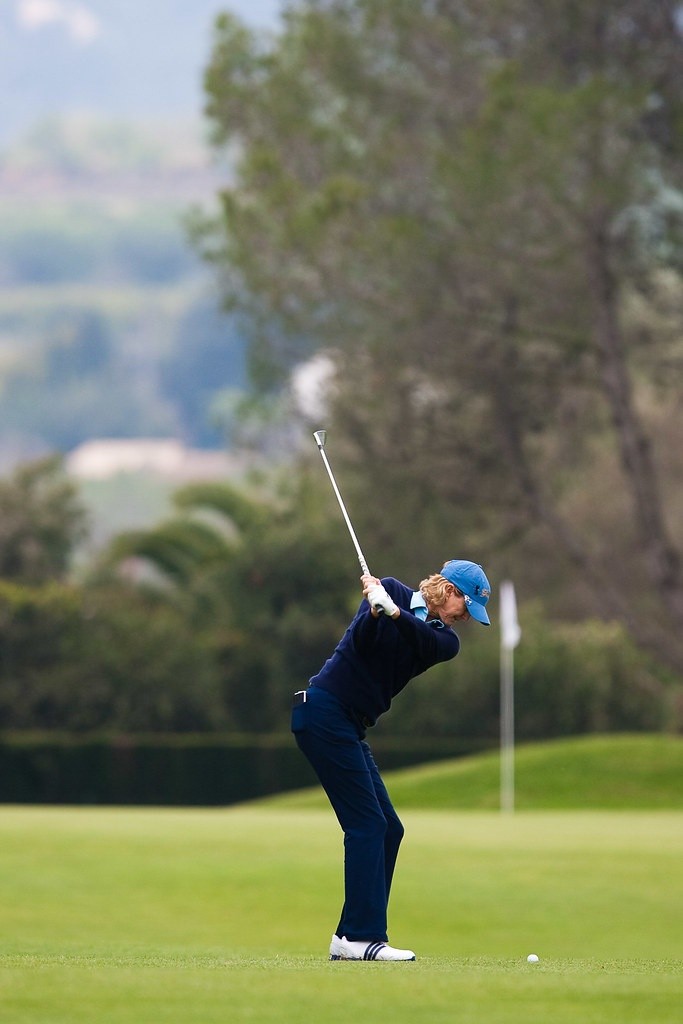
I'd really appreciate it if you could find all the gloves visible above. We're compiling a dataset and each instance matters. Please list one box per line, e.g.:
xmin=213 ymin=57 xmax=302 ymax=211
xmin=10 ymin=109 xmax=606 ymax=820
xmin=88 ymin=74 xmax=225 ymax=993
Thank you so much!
xmin=367 ymin=584 xmax=398 ymax=616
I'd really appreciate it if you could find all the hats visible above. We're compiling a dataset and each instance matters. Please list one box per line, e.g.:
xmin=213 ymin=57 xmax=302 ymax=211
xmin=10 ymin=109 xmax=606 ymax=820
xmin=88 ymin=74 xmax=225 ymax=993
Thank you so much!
xmin=440 ymin=560 xmax=491 ymax=626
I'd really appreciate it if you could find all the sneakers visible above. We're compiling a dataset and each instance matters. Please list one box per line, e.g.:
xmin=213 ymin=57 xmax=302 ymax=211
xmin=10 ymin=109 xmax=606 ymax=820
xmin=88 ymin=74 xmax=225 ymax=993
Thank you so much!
xmin=328 ymin=933 xmax=416 ymax=960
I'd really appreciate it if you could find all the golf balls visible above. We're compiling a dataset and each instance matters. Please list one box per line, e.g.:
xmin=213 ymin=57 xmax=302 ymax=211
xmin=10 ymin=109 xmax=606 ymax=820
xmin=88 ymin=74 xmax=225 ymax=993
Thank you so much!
xmin=526 ymin=954 xmax=539 ymax=964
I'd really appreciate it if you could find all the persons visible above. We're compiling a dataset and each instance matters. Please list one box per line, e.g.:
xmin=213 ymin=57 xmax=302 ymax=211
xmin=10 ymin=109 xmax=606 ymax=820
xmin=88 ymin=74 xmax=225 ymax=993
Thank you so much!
xmin=289 ymin=559 xmax=491 ymax=962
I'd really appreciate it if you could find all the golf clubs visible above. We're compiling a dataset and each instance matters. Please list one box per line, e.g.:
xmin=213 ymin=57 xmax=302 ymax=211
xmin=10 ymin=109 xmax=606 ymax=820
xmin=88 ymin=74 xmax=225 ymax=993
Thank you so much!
xmin=312 ymin=429 xmax=383 ymax=610
